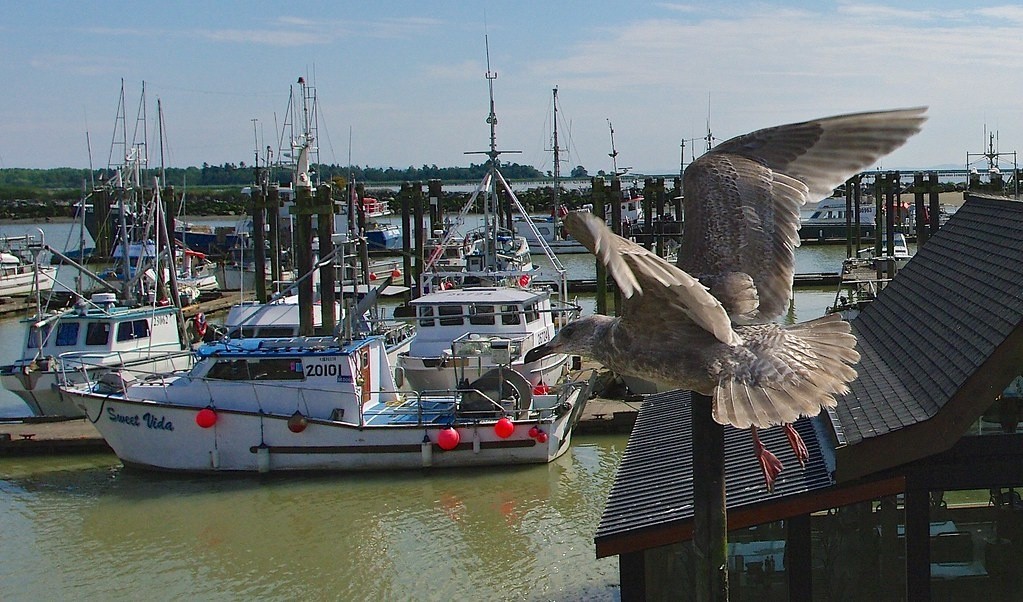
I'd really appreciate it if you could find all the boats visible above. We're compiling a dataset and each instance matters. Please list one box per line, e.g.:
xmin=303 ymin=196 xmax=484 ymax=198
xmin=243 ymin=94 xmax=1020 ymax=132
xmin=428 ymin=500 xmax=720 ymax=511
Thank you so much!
xmin=212 ymin=62 xmax=394 ymax=290
xmin=396 ymin=70 xmax=584 ymax=397
xmin=0 ymin=166 xmax=207 ymax=421
xmin=51 ymin=336 xmax=600 ymax=474
xmin=71 ymin=77 xmax=250 ymax=259
xmin=796 ymin=198 xmax=878 ymax=239
xmin=1 ymin=234 xmax=58 ymax=296
xmin=221 ymin=295 xmax=417 ymax=381
xmin=365 ymin=222 xmax=402 ymax=250
xmin=872 ymin=231 xmax=912 ymax=273
xmin=823 ymin=256 xmax=898 ymax=321
xmin=421 ymin=215 xmax=541 ymax=286
xmin=97 ymin=243 xmax=180 ymax=291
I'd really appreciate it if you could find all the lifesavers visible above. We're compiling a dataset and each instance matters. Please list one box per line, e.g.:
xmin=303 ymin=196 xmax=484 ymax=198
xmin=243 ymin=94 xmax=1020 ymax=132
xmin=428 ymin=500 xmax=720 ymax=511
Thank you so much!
xmin=196 ymin=312 xmax=207 ymax=334
xmin=463 ymin=232 xmax=470 ymax=254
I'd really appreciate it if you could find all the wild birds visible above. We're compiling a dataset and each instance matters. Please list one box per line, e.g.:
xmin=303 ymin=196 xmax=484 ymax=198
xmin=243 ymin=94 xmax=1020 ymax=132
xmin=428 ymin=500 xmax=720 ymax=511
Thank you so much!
xmin=524 ymin=106 xmax=932 ymax=491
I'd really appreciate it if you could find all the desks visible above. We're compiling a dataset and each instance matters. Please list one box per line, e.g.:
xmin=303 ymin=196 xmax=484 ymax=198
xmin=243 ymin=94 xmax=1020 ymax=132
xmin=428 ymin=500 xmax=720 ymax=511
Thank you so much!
xmin=930 ymin=558 xmax=991 ymax=582
xmin=727 ymin=542 xmax=785 ymax=573
xmin=876 ymin=520 xmax=959 ymax=540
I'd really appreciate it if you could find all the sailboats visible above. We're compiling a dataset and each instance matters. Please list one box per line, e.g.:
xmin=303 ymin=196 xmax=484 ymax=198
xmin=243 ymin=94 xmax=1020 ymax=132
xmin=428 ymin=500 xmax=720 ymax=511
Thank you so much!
xmin=529 ymin=89 xmax=591 ymax=254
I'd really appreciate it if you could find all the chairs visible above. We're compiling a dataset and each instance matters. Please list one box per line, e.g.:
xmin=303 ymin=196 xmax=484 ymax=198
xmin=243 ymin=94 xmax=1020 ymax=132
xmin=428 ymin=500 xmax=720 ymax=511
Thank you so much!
xmin=728 ymin=489 xmax=1022 ymax=602
